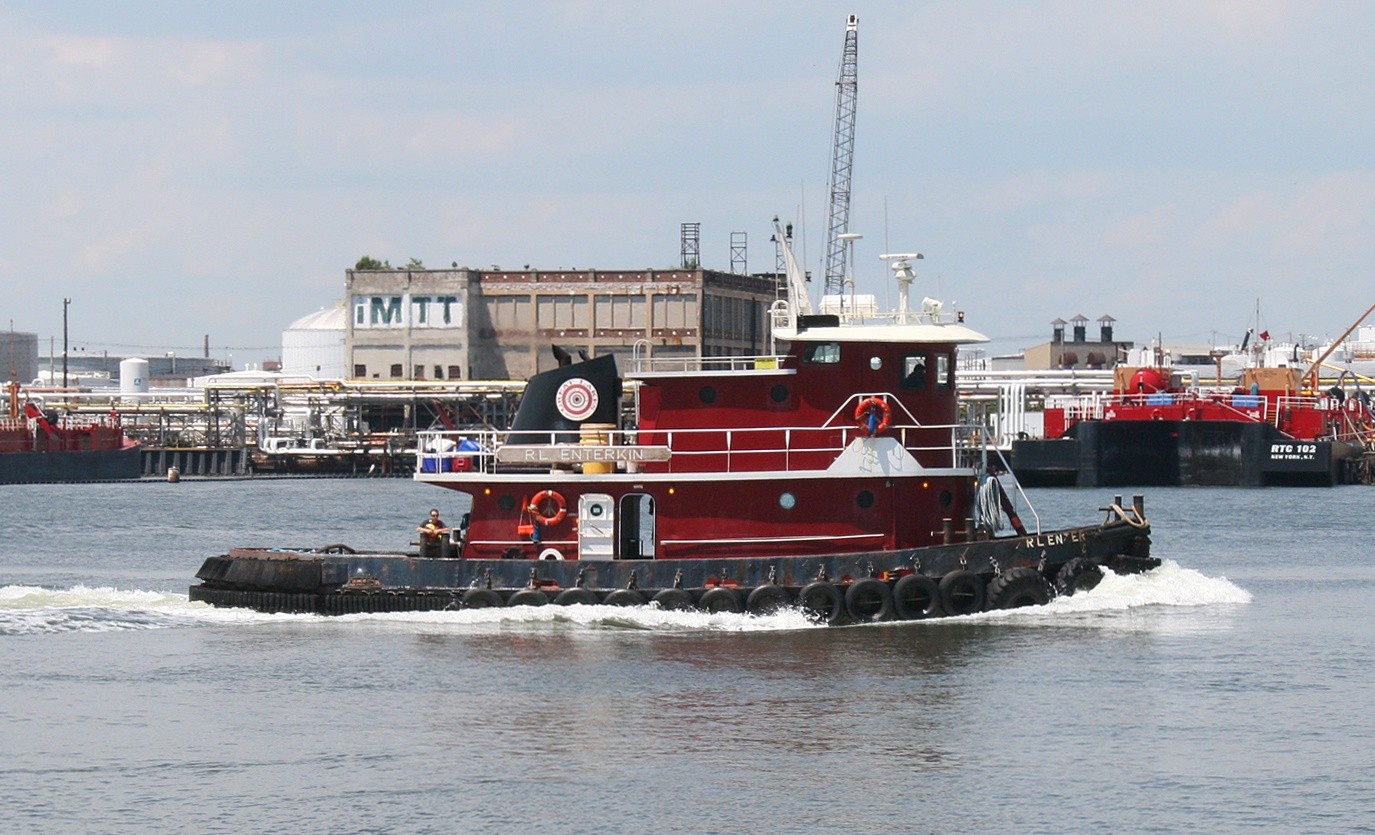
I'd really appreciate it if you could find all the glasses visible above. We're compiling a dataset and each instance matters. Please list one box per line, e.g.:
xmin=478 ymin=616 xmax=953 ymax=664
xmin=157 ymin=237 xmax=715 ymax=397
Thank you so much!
xmin=430 ymin=515 xmax=439 ymax=517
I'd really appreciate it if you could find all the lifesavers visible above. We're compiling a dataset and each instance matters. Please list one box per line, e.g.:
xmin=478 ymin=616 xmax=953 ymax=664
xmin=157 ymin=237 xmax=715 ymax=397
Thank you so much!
xmin=798 ymin=582 xmax=843 ymax=625
xmin=462 ymin=589 xmax=504 ymax=608
xmin=747 ymin=584 xmax=794 ymax=613
xmin=530 ymin=489 xmax=567 ymax=527
xmin=651 ymin=588 xmax=692 ymax=611
xmin=603 ymin=589 xmax=647 ymax=607
xmin=893 ymin=574 xmax=939 ymax=619
xmin=846 ymin=577 xmax=892 ymax=624
xmin=985 ymin=567 xmax=1056 ymax=611
xmin=939 ymin=569 xmax=985 ymax=616
xmin=507 ymin=590 xmax=549 ymax=607
xmin=855 ymin=397 xmax=892 ymax=435
xmin=699 ymin=587 xmax=743 ymax=613
xmin=554 ymin=587 xmax=598 ymax=607
xmin=1056 ymin=557 xmax=1103 ymax=597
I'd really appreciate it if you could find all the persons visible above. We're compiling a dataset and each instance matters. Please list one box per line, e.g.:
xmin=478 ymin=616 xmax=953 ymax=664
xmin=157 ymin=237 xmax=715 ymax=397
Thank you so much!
xmin=904 ymin=362 xmax=925 ymax=391
xmin=419 ymin=509 xmax=452 ymax=559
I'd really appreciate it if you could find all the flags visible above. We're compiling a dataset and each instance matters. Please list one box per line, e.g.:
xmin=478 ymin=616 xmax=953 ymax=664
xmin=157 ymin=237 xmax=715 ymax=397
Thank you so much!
xmin=1259 ymin=330 xmax=1270 ymax=341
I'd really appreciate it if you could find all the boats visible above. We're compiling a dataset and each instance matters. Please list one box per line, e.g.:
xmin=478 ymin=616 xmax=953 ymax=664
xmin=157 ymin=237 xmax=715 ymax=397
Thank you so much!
xmin=0 ymin=320 xmax=142 ymax=484
xmin=185 ymin=218 xmax=1166 ymax=626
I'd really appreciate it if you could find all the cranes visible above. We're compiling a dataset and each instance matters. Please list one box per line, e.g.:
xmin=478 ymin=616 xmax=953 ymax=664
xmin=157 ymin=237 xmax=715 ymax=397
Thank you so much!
xmin=819 ymin=14 xmax=861 ymax=294
